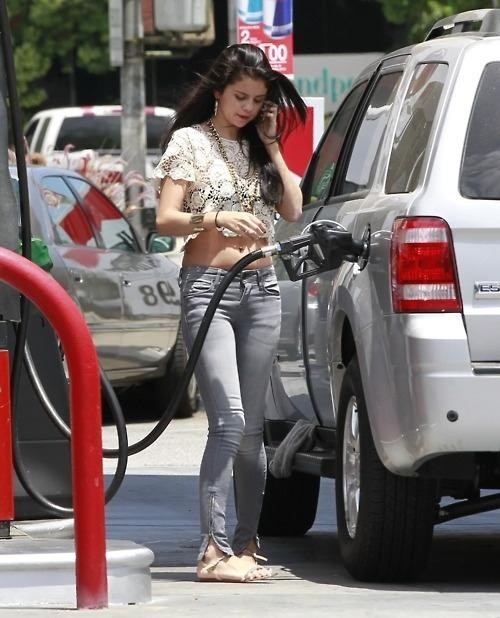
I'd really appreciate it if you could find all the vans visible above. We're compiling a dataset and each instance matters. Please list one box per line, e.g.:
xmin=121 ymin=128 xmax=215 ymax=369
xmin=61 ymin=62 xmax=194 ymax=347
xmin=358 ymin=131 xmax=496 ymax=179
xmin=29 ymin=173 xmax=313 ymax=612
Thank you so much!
xmin=24 ymin=101 xmax=186 ymax=216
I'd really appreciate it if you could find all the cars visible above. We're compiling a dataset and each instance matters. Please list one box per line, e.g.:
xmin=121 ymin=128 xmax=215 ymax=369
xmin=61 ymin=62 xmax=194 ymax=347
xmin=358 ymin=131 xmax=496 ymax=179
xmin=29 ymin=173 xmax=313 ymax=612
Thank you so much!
xmin=5 ymin=163 xmax=202 ymax=419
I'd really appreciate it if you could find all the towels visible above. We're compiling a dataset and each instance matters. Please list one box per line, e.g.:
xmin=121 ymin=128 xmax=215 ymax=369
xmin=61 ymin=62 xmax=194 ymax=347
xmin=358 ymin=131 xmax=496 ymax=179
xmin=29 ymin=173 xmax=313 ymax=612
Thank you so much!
xmin=266 ymin=417 xmax=317 ymax=480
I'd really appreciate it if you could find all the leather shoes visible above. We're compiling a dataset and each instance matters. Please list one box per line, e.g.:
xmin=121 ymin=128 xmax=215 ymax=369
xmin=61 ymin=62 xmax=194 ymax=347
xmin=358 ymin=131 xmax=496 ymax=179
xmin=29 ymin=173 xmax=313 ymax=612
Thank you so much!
xmin=196 ymin=549 xmax=272 ymax=582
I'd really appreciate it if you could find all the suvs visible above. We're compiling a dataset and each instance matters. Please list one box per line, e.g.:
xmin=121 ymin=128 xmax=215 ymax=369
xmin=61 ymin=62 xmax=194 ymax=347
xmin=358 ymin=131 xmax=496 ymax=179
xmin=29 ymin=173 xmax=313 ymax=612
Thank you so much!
xmin=234 ymin=7 xmax=500 ymax=588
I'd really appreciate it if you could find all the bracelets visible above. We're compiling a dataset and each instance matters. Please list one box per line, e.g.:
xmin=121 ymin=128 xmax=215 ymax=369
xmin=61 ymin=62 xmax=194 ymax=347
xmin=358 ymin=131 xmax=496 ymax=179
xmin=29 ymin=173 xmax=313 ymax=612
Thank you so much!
xmin=190 ymin=212 xmax=208 ymax=234
xmin=215 ymin=211 xmax=221 ymax=228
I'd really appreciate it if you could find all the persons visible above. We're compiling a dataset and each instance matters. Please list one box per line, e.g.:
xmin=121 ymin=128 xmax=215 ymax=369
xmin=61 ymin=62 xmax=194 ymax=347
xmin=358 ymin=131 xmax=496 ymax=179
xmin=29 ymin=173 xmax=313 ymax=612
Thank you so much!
xmin=146 ymin=44 xmax=307 ymax=583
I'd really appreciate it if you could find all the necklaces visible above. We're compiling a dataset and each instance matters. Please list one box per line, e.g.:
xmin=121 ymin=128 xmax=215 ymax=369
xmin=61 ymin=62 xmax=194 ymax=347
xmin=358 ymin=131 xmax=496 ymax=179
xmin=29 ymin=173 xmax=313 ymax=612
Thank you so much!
xmin=206 ymin=118 xmax=262 ymax=215
